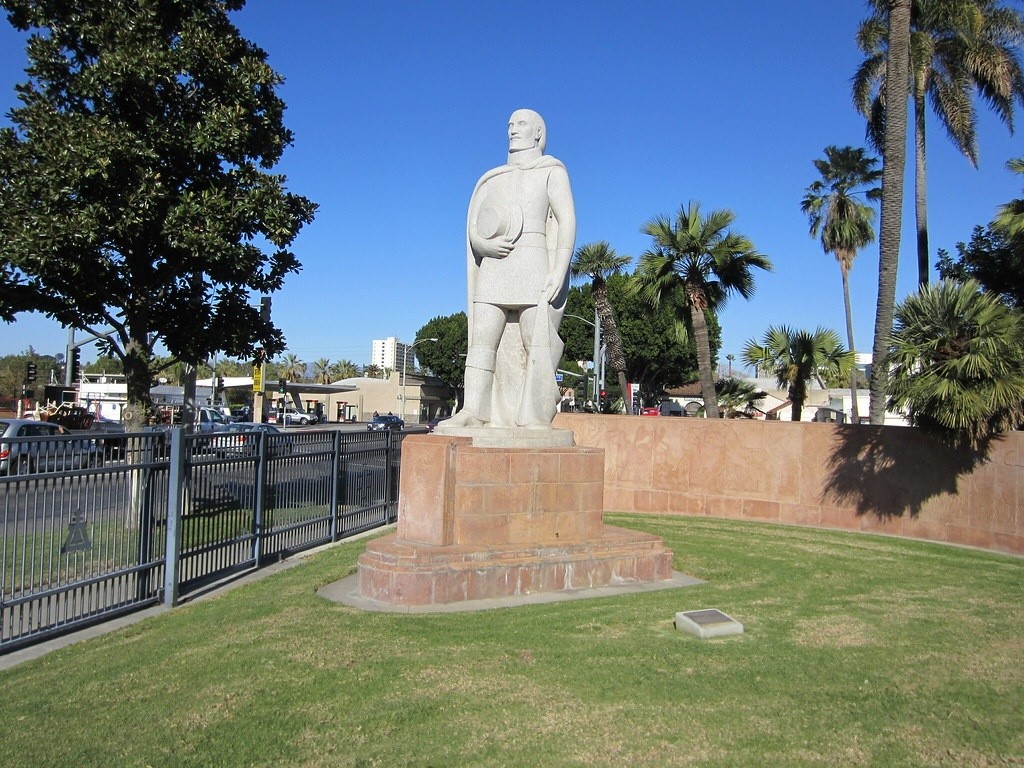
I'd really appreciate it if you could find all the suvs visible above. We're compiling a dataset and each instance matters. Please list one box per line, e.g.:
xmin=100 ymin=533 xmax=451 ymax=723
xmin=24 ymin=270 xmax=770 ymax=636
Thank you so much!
xmin=278 ymin=407 xmax=319 ymax=426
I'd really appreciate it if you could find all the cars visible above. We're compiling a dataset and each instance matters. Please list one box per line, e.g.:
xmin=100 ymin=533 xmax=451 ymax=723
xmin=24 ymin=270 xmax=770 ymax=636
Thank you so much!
xmin=0 ymin=418 xmax=107 ymax=477
xmin=210 ymin=422 xmax=293 ymax=461
xmin=425 ymin=418 xmax=447 ymax=435
xmin=366 ymin=416 xmax=404 ymax=432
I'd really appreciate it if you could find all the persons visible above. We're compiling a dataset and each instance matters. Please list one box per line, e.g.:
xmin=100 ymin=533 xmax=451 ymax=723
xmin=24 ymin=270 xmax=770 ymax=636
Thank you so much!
xmin=388 ymin=411 xmax=393 ymax=415
xmin=434 ymin=107 xmax=577 ymax=431
xmin=373 ymin=411 xmax=379 ymax=418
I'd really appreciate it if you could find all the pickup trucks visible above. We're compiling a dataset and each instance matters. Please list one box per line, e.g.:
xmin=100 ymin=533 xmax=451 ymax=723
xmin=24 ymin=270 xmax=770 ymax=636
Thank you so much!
xmin=156 ymin=407 xmax=235 ymax=452
xmin=68 ymin=400 xmax=165 ymax=458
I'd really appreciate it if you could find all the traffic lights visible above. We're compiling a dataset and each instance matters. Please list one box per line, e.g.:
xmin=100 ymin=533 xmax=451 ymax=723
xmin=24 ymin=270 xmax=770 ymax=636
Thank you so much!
xmin=600 ymin=390 xmax=606 ymax=408
xmin=279 ymin=378 xmax=287 ymax=393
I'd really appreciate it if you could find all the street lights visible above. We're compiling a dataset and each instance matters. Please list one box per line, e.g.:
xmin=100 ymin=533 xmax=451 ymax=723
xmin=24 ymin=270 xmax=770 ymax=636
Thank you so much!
xmin=400 ymin=338 xmax=438 ymax=420
